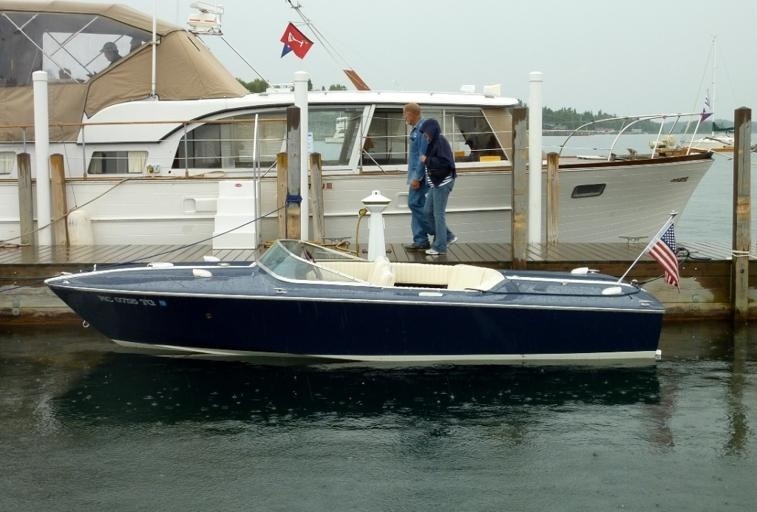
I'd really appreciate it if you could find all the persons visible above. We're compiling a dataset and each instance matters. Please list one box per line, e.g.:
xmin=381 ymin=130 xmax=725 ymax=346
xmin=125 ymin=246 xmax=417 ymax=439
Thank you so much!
xmin=85 ymin=43 xmax=123 ymax=75
xmin=419 ymin=119 xmax=457 ymax=256
xmin=130 ymin=39 xmax=140 ymax=51
xmin=403 ymin=103 xmax=458 ymax=249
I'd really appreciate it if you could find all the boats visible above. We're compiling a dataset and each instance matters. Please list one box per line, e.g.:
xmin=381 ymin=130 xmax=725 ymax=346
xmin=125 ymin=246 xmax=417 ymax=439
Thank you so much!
xmin=0 ymin=2 xmax=713 ymax=247
xmin=42 ymin=208 xmax=678 ymax=367
xmin=38 ymin=351 xmax=661 ymax=444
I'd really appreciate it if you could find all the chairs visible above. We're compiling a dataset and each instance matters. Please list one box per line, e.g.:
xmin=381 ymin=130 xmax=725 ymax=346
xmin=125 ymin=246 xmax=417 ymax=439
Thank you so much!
xmin=440 ymin=262 xmax=509 ymax=298
xmin=368 ymin=253 xmax=396 ymax=287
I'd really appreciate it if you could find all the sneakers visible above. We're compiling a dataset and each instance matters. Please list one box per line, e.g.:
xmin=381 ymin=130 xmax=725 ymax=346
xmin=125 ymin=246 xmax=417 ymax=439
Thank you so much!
xmin=447 ymin=234 xmax=458 ymax=248
xmin=404 ymin=242 xmax=431 ymax=249
xmin=425 ymin=247 xmax=448 ymax=255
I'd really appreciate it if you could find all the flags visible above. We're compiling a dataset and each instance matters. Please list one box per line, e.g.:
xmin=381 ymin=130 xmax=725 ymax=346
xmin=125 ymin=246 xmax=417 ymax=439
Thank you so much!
xmin=701 ymin=95 xmax=712 ymax=125
xmin=650 ymin=222 xmax=681 ymax=294
xmin=280 ymin=23 xmax=312 ymax=59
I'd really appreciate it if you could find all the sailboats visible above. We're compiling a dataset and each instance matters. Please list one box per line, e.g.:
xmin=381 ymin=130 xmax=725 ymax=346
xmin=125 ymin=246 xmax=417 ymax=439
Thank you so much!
xmin=648 ymin=33 xmax=756 ymax=160
